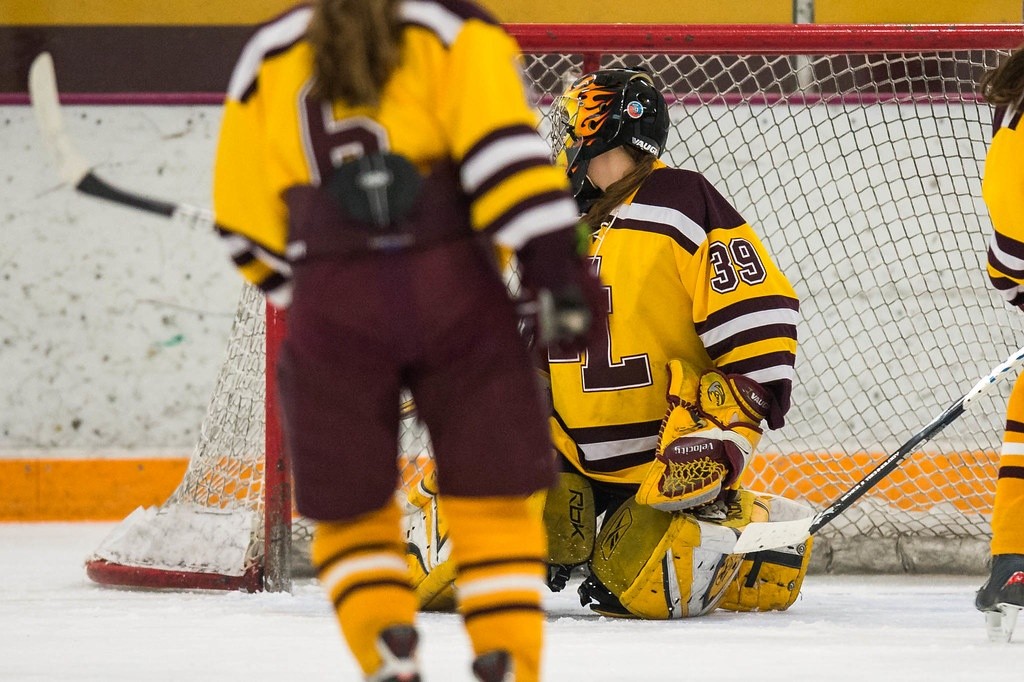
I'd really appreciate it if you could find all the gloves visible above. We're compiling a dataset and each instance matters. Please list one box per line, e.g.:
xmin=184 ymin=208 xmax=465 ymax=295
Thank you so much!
xmin=511 ymin=226 xmax=606 ymax=361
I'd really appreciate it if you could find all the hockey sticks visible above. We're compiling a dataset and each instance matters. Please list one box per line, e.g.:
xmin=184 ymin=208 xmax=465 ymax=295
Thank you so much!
xmin=30 ymin=48 xmax=220 ymax=237
xmin=731 ymin=340 xmax=1024 ymax=555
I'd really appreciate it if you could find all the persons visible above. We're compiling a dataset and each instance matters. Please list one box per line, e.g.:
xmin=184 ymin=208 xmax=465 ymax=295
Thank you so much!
xmin=976 ymin=49 xmax=1024 ymax=644
xmin=213 ymin=0 xmax=610 ymax=682
xmin=399 ymin=70 xmax=817 ymax=622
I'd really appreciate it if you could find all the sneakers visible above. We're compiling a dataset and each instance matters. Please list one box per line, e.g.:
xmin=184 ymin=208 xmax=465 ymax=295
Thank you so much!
xmin=975 ymin=553 xmax=1024 ymax=643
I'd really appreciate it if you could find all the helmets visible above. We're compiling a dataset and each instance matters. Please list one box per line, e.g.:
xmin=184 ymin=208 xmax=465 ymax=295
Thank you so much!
xmin=548 ymin=66 xmax=670 ymax=198
